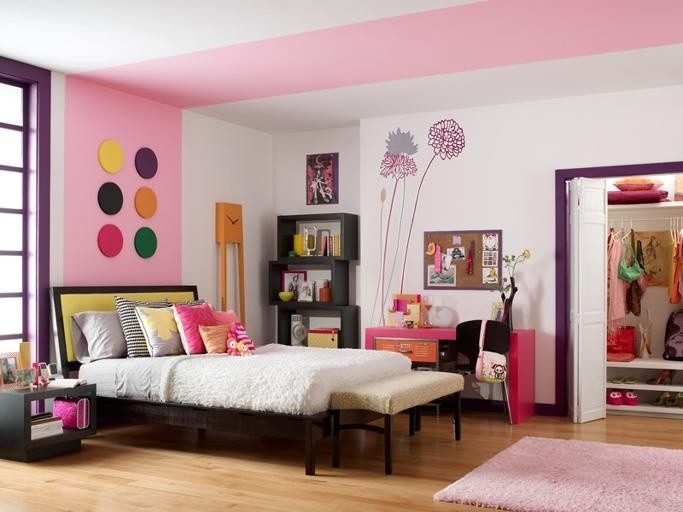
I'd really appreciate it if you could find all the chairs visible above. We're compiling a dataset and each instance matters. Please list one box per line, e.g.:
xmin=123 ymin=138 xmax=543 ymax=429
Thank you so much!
xmin=451 ymin=319 xmax=512 ymax=424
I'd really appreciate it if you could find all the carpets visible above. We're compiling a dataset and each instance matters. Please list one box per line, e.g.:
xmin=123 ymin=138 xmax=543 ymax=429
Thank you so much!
xmin=434 ymin=434 xmax=683 ymax=510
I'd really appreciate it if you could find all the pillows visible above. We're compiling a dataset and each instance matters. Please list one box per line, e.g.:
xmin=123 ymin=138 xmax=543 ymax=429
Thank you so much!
xmin=212 ymin=308 xmax=239 ymax=326
xmin=608 ymin=189 xmax=670 ymax=204
xmin=110 ymin=291 xmax=174 ymax=357
xmin=610 ymin=177 xmax=667 ymax=190
xmin=172 ymin=300 xmax=215 ymax=354
xmin=67 ymin=307 xmax=127 ymax=362
xmin=185 ymin=299 xmax=204 ymax=306
xmin=133 ymin=302 xmax=185 ymax=358
xmin=196 ymin=321 xmax=235 ymax=353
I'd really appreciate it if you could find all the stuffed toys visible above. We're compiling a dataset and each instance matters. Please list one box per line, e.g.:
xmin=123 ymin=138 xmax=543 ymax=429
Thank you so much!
xmin=236 ymin=340 xmax=251 ymax=356
xmin=231 ymin=321 xmax=255 ymax=354
xmin=226 ymin=333 xmax=240 ymax=356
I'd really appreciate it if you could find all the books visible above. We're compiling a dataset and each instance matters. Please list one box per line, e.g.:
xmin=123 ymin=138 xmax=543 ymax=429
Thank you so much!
xmin=311 ymin=228 xmax=341 ymax=256
xmin=388 ymin=292 xmax=433 ymax=328
xmin=48 ymin=376 xmax=88 ymax=389
xmin=30 ymin=411 xmax=66 ymax=440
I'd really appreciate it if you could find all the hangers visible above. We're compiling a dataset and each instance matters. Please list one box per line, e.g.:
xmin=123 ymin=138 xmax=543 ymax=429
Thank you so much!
xmin=609 ymin=217 xmax=683 ymax=245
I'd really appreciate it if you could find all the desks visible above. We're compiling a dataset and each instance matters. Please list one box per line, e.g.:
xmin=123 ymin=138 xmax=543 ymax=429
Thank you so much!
xmin=364 ymin=326 xmax=536 ymax=425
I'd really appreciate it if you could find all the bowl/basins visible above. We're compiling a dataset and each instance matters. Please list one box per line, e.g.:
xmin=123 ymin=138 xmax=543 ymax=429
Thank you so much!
xmin=278 ymin=292 xmax=294 ymax=302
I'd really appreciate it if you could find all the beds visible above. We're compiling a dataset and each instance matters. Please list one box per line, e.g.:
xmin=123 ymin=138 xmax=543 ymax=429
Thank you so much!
xmin=49 ymin=283 xmax=417 ymax=477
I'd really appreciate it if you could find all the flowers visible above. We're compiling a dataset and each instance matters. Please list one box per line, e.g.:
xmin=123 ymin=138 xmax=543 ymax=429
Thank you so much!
xmin=372 ymin=116 xmax=466 ymax=324
xmin=487 ymin=246 xmax=531 ymax=294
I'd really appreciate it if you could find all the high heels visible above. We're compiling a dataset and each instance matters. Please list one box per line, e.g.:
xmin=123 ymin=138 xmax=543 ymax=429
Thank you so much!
xmin=653 ymin=392 xmax=670 ymax=407
xmin=647 ymin=371 xmax=669 ymax=384
xmin=668 ymin=392 xmax=683 ymax=409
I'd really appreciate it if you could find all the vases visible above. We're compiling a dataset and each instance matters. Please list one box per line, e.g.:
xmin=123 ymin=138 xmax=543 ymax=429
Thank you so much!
xmin=500 ymin=278 xmax=518 ymax=330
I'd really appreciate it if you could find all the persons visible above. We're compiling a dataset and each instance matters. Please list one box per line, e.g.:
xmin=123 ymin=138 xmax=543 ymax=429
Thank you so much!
xmin=297 ymin=282 xmax=308 ymax=302
xmin=2 ymin=359 xmax=16 ymax=384
xmin=288 ymin=274 xmax=299 ymax=299
xmin=305 ymin=288 xmax=312 ymax=302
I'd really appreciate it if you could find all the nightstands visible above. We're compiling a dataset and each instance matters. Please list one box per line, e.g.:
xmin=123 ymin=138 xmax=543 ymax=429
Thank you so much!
xmin=1 ymin=380 xmax=97 ymax=463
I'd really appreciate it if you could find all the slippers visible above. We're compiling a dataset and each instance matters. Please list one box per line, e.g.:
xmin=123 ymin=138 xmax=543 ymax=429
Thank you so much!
xmin=622 ymin=391 xmax=637 ymax=404
xmin=612 ymin=375 xmax=623 ymax=383
xmin=605 ymin=390 xmax=624 ymax=404
xmin=625 ymin=375 xmax=637 ymax=384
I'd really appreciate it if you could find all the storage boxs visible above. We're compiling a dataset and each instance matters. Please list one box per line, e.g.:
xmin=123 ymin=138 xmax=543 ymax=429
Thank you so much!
xmin=306 ymin=328 xmax=340 ymax=348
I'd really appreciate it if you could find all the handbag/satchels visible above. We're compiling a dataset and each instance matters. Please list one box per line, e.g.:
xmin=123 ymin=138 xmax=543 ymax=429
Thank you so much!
xmin=638 ymin=274 xmax=647 ymax=292
xmin=619 ymin=237 xmax=643 ymax=281
xmin=630 ymin=283 xmax=641 ymax=315
xmin=475 ymin=318 xmax=507 ymax=383
xmin=639 ymin=312 xmax=654 ymax=358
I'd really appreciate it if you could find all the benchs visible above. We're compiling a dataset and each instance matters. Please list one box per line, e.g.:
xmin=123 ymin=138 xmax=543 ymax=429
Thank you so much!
xmin=328 ymin=371 xmax=465 ymax=474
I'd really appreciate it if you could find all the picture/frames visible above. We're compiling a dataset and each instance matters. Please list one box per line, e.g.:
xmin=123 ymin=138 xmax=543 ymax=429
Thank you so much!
xmin=296 ymin=278 xmax=314 ymax=302
xmin=281 ymin=271 xmax=308 ymax=301
xmin=423 ymin=230 xmax=503 ymax=290
xmin=0 ymin=352 xmax=23 ymax=390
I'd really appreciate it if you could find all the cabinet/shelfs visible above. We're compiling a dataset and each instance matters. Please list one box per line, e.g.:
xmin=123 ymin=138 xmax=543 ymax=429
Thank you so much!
xmin=606 ymin=172 xmax=683 ymax=418
xmin=267 ymin=213 xmax=360 ymax=348
